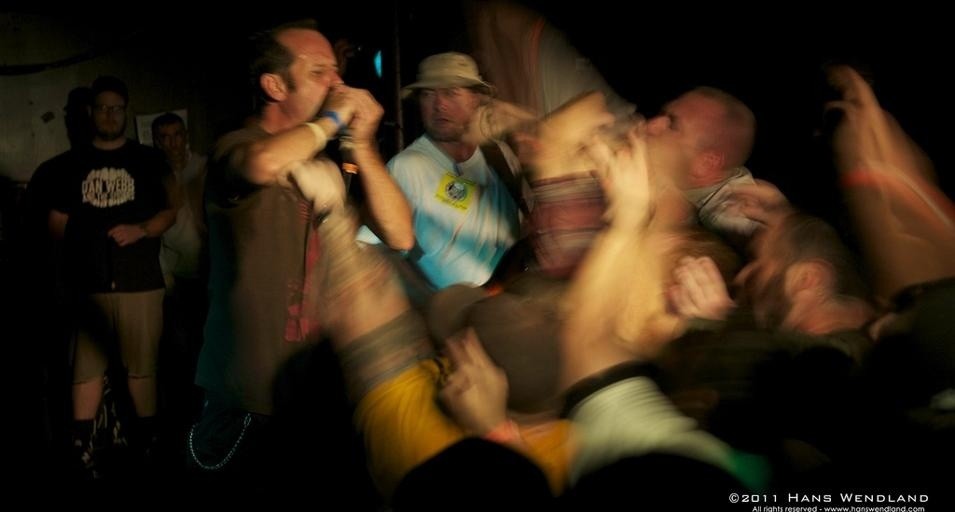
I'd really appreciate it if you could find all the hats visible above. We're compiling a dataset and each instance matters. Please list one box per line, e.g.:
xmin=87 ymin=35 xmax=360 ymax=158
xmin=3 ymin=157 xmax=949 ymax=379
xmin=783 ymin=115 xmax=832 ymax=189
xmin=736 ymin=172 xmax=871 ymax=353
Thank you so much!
xmin=401 ymin=52 xmax=499 ymax=99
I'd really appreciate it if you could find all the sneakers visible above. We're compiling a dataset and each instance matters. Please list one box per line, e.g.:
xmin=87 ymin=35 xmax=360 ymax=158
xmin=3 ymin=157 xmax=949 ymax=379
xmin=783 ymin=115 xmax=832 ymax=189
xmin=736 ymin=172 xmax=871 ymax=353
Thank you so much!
xmin=63 ymin=439 xmax=94 ymax=470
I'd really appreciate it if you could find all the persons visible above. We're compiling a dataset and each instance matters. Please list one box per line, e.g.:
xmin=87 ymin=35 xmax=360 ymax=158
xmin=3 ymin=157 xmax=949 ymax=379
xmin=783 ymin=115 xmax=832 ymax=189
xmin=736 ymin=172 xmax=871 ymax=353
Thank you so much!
xmin=140 ymin=109 xmax=215 ymax=408
xmin=26 ymin=70 xmax=184 ymax=475
xmin=183 ymin=22 xmax=417 ymax=510
xmin=268 ymin=47 xmax=955 ymax=512
